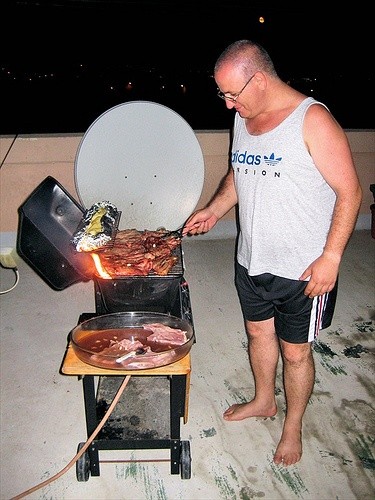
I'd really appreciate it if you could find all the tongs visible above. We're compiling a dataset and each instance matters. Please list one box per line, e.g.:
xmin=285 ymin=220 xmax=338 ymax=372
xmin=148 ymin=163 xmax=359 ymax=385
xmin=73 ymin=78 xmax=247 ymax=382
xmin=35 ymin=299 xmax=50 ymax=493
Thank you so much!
xmin=162 ymin=221 xmax=201 ymax=240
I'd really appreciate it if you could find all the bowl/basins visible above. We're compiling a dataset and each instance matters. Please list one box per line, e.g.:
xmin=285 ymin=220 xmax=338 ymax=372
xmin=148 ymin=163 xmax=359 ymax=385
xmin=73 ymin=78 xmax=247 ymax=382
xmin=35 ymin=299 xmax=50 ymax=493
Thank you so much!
xmin=70 ymin=311 xmax=194 ymax=371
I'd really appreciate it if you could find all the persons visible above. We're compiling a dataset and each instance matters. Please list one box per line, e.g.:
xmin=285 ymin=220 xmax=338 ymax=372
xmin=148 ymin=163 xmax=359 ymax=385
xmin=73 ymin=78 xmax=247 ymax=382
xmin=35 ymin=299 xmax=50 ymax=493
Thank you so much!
xmin=182 ymin=40 xmax=363 ymax=466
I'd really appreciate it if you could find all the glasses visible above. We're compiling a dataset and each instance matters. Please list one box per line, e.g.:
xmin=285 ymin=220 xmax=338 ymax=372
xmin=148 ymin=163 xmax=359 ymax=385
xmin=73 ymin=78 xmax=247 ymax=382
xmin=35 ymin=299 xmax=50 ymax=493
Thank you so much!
xmin=216 ymin=75 xmax=257 ymax=104
